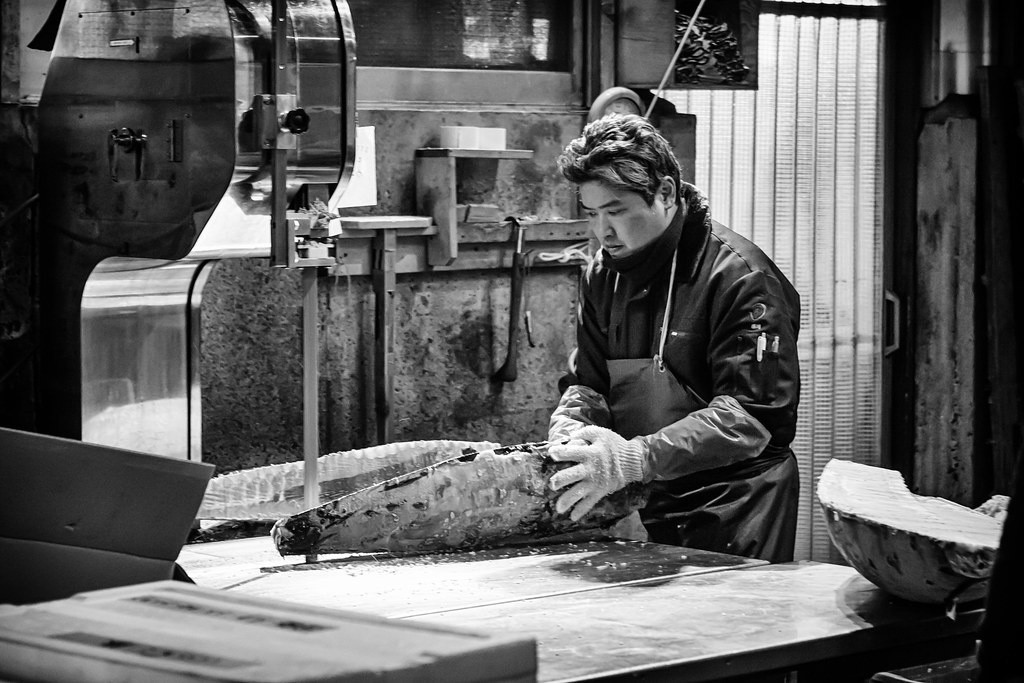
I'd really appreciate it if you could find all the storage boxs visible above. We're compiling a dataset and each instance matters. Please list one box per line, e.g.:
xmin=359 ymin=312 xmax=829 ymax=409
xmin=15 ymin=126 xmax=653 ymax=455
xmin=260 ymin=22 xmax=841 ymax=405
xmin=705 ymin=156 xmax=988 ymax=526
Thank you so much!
xmin=0 ymin=579 xmax=542 ymax=683
xmin=1 ymin=427 xmax=216 ymax=605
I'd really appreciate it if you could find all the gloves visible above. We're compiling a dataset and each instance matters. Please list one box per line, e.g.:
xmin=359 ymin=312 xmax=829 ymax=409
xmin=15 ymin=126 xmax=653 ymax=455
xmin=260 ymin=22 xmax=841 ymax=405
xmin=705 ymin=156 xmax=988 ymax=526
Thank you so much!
xmin=548 ymin=424 xmax=645 ymax=521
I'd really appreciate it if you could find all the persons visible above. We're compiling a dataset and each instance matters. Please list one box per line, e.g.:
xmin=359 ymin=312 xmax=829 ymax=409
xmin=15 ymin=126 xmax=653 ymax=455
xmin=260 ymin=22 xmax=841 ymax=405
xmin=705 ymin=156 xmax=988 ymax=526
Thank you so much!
xmin=544 ymin=115 xmax=803 ymax=563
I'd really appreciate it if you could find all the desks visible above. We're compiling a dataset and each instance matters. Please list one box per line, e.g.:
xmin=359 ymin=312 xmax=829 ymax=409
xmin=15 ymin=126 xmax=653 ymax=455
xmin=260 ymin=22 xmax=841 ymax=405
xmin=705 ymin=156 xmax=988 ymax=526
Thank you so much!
xmin=165 ymin=533 xmax=990 ymax=682
